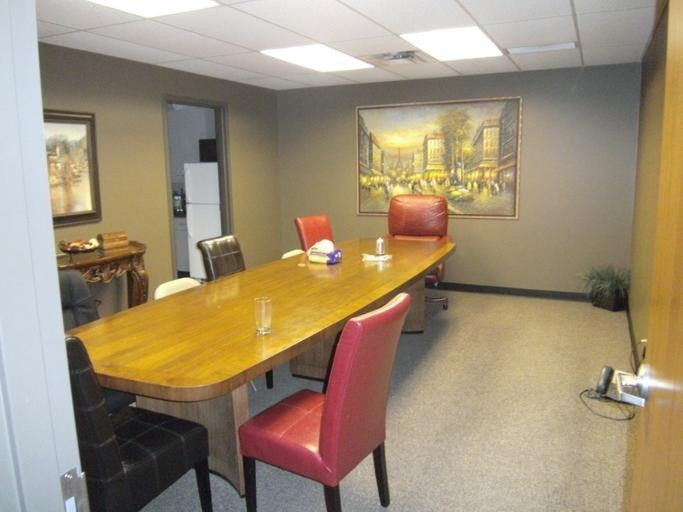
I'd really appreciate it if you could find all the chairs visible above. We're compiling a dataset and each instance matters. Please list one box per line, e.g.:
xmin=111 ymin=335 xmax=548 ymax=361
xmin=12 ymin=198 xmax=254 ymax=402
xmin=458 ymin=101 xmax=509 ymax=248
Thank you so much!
xmin=154 ymin=277 xmax=200 ymax=302
xmin=281 ymin=249 xmax=304 ymax=260
xmin=197 ymin=235 xmax=273 ymax=389
xmin=294 ymin=215 xmax=336 ymax=253
xmin=380 ymin=194 xmax=449 ymax=311
xmin=66 ymin=334 xmax=213 ymax=512
xmin=238 ymin=293 xmax=412 ymax=512
xmin=58 ymin=269 xmax=99 ymax=333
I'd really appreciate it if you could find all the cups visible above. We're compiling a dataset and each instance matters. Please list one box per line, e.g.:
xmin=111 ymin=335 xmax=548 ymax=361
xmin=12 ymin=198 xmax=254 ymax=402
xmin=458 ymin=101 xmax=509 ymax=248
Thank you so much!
xmin=253 ymin=297 xmax=273 ymax=336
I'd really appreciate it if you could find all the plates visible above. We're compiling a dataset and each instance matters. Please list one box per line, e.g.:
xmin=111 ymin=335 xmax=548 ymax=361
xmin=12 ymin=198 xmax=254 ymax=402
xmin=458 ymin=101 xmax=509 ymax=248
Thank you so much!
xmin=58 ymin=241 xmax=100 ymax=254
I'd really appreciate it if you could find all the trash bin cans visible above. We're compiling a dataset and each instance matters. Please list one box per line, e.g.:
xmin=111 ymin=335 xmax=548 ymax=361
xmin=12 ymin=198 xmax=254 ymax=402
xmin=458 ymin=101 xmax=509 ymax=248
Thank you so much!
xmin=86 ymin=270 xmax=129 ymax=318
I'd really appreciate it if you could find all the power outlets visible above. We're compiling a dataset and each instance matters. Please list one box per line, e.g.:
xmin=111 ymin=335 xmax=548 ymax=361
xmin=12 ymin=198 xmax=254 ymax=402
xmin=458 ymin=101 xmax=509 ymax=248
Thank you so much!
xmin=642 ymin=343 xmax=647 ymax=360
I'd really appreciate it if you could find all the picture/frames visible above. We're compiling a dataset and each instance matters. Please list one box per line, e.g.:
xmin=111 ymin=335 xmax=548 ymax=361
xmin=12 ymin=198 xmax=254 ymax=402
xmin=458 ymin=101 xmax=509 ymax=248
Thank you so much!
xmin=356 ymin=96 xmax=522 ymax=220
xmin=43 ymin=109 xmax=102 ymax=229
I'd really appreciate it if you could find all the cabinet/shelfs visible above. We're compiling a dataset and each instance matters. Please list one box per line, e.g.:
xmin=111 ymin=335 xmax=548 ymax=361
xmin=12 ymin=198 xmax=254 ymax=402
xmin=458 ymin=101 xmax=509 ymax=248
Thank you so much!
xmin=173 ymin=217 xmax=190 ymax=273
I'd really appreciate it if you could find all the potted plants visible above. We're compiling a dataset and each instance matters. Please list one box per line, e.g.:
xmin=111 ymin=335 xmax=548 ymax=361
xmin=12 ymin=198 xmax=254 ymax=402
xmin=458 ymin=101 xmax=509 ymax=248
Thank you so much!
xmin=576 ymin=263 xmax=632 ymax=313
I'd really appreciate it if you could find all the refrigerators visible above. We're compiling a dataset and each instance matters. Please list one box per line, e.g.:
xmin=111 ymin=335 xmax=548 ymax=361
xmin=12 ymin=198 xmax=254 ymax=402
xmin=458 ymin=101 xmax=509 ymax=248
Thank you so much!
xmin=184 ymin=162 xmax=223 ymax=281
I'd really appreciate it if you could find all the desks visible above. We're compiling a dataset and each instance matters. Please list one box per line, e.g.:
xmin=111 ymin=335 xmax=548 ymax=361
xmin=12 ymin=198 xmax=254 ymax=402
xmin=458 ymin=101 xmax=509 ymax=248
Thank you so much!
xmin=66 ymin=238 xmax=457 ymax=498
xmin=56 ymin=240 xmax=148 ymax=309
